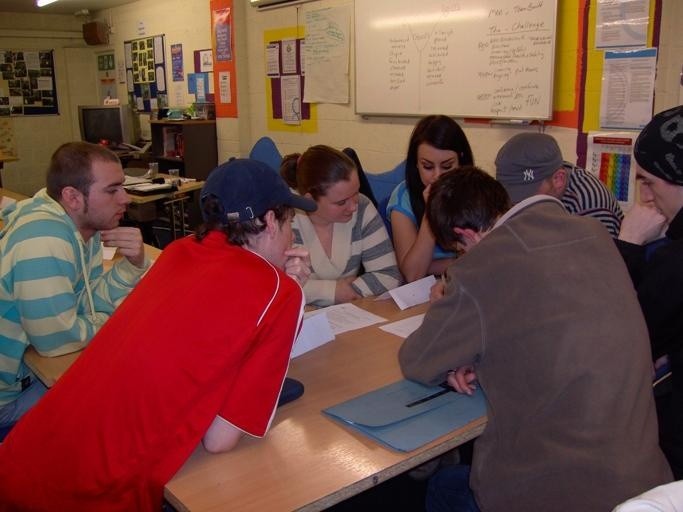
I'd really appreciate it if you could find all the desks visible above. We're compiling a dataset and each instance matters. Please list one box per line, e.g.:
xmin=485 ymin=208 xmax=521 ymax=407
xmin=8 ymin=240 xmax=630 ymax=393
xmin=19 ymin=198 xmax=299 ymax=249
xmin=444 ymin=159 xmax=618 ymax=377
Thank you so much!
xmin=0 ymin=187 xmax=163 ymax=390
xmin=121 ymin=166 xmax=208 ymax=241
xmin=163 ymin=275 xmax=487 ymax=511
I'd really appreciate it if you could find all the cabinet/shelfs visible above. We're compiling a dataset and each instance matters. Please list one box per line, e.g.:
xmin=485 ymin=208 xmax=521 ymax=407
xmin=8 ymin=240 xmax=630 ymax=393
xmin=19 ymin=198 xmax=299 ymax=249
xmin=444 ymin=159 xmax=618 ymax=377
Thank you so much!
xmin=150 ymin=118 xmax=217 ymax=224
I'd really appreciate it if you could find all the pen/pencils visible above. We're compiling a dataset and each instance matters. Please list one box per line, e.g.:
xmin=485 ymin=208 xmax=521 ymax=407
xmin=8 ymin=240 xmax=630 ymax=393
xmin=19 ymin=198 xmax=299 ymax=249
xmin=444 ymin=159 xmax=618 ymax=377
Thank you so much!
xmin=441 ymin=268 xmax=448 ymax=289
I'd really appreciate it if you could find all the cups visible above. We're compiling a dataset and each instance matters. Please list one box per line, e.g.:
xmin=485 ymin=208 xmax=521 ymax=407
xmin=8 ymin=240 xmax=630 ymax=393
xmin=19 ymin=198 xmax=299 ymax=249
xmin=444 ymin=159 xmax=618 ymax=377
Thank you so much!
xmin=168 ymin=169 xmax=179 ymax=184
xmin=168 ymin=110 xmax=181 ymax=119
xmin=148 ymin=162 xmax=158 ymax=179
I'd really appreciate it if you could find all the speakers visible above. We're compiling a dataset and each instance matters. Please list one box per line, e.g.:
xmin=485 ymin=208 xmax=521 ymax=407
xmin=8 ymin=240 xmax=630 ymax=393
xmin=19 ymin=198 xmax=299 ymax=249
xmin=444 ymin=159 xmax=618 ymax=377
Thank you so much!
xmin=82 ymin=22 xmax=110 ymax=45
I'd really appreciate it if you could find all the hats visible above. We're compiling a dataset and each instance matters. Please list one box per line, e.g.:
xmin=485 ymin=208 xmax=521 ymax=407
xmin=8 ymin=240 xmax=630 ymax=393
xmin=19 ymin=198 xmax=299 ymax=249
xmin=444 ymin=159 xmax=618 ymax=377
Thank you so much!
xmin=199 ymin=157 xmax=317 ymax=226
xmin=634 ymin=105 xmax=683 ymax=186
xmin=494 ymin=132 xmax=563 ymax=203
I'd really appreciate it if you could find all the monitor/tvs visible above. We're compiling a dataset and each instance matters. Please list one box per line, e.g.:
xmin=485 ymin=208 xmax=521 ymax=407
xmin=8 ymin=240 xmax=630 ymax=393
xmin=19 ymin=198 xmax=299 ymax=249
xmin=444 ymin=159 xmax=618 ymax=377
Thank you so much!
xmin=78 ymin=104 xmax=141 ymax=153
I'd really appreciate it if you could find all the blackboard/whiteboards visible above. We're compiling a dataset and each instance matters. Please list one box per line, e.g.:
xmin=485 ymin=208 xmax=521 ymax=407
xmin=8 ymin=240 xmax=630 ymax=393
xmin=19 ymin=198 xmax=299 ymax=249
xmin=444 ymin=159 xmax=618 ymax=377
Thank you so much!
xmin=354 ymin=0 xmax=557 ymax=126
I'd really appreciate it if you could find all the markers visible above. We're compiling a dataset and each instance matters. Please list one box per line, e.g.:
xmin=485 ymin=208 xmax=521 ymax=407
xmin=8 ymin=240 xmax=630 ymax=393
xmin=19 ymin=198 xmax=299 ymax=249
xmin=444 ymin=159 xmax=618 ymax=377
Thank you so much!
xmin=491 ymin=120 xmax=529 ymax=124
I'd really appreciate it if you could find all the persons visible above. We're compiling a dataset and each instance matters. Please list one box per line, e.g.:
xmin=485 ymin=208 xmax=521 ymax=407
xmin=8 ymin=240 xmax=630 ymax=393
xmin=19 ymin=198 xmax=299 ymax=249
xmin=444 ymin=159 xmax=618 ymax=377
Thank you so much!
xmin=1 ymin=156 xmax=317 ymax=511
xmin=278 ymin=144 xmax=404 ymax=308
xmin=2 ymin=141 xmax=157 ymax=431
xmin=385 ymin=114 xmax=474 ymax=284
xmin=495 ymin=131 xmax=621 ymax=243
xmin=612 ymin=105 xmax=681 ymax=480
xmin=397 ymin=166 xmax=673 ymax=512
